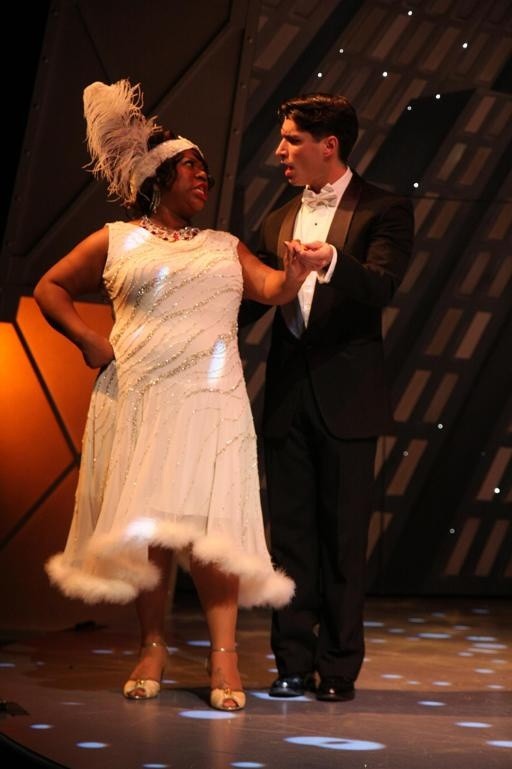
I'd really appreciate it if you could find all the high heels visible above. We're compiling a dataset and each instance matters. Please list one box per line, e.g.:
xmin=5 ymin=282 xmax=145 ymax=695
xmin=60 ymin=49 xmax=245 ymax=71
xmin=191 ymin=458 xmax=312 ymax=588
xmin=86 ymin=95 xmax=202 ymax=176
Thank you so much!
xmin=122 ymin=640 xmax=169 ymax=700
xmin=205 ymin=646 xmax=246 ymax=710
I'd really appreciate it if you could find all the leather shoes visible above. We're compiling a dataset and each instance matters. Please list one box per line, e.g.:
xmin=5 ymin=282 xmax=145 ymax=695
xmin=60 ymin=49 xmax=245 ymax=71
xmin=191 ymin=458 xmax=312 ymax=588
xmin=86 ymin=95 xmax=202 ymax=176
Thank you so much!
xmin=269 ymin=670 xmax=316 ymax=695
xmin=316 ymin=675 xmax=355 ymax=701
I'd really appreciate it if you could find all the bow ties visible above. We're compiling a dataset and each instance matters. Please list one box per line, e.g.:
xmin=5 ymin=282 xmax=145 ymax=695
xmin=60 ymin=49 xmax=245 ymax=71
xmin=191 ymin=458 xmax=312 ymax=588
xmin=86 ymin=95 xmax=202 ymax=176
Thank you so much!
xmin=301 ymin=184 xmax=337 ymax=211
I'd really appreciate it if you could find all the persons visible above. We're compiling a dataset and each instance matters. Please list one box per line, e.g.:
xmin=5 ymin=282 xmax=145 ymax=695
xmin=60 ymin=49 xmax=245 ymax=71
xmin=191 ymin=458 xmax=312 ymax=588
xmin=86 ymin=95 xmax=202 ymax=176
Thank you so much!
xmin=34 ymin=129 xmax=319 ymax=712
xmin=242 ymin=87 xmax=413 ymax=704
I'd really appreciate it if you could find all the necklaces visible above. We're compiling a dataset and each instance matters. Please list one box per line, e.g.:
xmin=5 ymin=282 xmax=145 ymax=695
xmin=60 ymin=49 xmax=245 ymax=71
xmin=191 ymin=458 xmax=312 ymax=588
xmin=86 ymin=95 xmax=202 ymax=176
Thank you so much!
xmin=139 ymin=210 xmax=204 ymax=242
